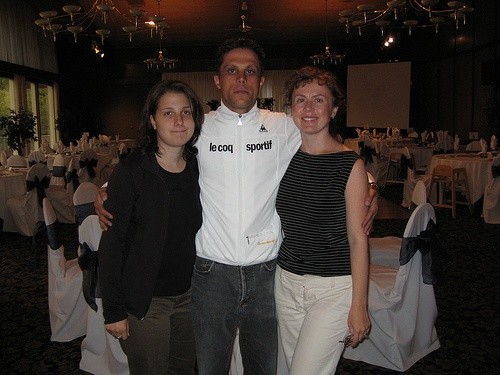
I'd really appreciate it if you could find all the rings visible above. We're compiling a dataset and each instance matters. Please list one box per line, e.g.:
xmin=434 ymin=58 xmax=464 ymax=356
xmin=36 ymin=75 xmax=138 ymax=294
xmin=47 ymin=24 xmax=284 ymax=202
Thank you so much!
xmin=117 ymin=335 xmax=122 ymax=338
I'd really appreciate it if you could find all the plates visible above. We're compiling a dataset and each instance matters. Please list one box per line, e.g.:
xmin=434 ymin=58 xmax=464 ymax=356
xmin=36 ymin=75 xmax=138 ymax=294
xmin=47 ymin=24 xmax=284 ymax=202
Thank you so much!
xmin=353 ymin=137 xmax=494 ymax=162
xmin=0 ymin=166 xmax=13 ymax=175
xmin=55 ymin=140 xmax=120 ymax=156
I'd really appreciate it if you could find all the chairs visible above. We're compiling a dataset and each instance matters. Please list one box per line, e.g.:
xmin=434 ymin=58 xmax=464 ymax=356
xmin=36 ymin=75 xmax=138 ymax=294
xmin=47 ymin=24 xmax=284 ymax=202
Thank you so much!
xmin=0 ymin=125 xmax=500 ymax=375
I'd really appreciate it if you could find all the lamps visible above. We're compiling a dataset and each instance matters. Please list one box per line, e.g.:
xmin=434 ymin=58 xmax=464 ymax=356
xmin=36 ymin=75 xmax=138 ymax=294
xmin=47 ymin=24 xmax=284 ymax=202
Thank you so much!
xmin=33 ymin=0 xmax=474 ymax=70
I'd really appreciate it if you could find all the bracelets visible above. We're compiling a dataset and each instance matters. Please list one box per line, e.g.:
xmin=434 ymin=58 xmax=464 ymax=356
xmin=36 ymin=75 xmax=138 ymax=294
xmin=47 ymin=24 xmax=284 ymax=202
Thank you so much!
xmin=368 ymin=182 xmax=380 ymax=191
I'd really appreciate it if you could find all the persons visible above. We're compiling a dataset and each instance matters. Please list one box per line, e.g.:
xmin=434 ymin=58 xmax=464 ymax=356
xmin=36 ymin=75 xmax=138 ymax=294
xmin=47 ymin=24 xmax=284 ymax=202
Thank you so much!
xmin=272 ymin=67 xmax=372 ymax=374
xmin=94 ymin=38 xmax=378 ymax=375
xmin=98 ymin=80 xmax=199 ymax=375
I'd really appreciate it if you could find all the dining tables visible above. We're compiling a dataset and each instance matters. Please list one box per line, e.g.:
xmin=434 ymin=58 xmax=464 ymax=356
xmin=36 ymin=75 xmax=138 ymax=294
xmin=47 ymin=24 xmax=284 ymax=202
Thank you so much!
xmin=44 ymin=146 xmax=116 ymax=172
xmin=425 ymin=152 xmax=497 ymax=213
xmin=0 ymin=167 xmax=30 ymax=233
xmin=344 ymin=138 xmax=386 ymax=156
